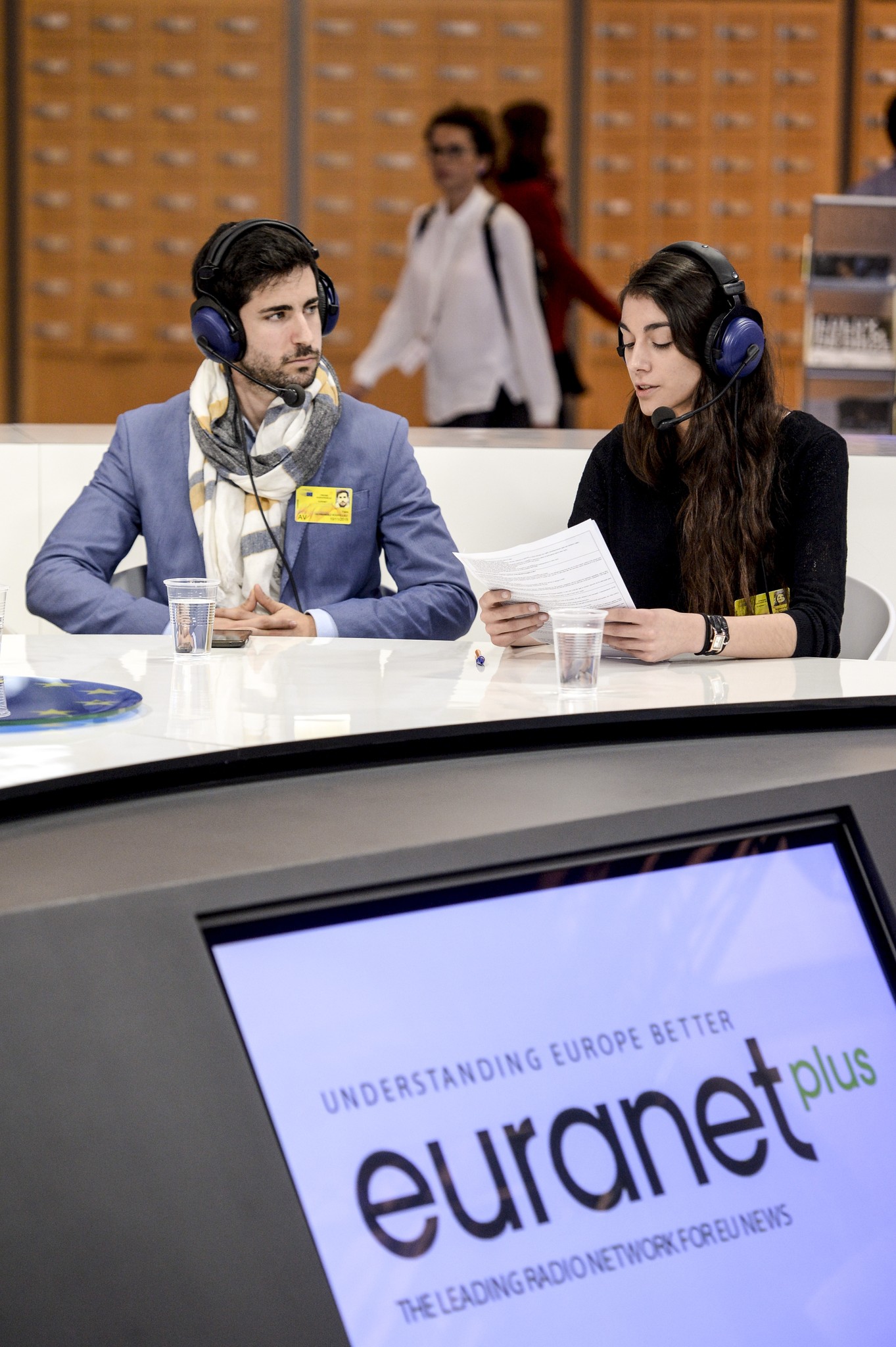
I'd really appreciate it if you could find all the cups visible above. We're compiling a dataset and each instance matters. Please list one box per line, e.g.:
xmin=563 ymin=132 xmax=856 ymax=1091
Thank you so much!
xmin=163 ymin=578 xmax=221 ymax=659
xmin=0 ymin=676 xmax=11 ymax=718
xmin=548 ymin=608 xmax=609 ymax=696
xmin=0 ymin=585 xmax=9 ymax=644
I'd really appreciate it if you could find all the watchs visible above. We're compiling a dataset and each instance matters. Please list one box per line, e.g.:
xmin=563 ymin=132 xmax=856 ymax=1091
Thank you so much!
xmin=705 ymin=615 xmax=726 ymax=656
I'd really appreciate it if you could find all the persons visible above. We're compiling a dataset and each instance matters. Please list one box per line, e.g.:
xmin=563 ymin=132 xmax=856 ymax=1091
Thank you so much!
xmin=833 ymin=94 xmax=896 ymax=437
xmin=774 ymin=592 xmax=786 ymax=607
xmin=335 ymin=491 xmax=349 ymax=508
xmin=25 ymin=217 xmax=478 ymax=641
xmin=479 ymin=240 xmax=850 ymax=663
xmin=346 ymin=108 xmax=561 ymax=428
xmin=480 ymin=100 xmax=623 ymax=430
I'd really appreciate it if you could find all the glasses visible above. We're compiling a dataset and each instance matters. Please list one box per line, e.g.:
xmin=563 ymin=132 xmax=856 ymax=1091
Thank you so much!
xmin=429 ymin=146 xmax=476 ymax=157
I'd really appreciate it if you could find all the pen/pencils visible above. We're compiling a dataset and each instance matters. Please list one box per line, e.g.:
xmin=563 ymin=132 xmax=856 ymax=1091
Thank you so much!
xmin=475 ymin=649 xmax=485 ymax=665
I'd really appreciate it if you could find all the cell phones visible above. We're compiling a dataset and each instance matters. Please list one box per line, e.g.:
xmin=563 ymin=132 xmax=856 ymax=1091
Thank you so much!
xmin=211 ymin=629 xmax=251 ymax=648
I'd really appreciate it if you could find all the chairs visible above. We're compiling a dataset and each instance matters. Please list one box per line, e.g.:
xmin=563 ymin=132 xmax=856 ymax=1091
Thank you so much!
xmin=835 ymin=575 xmax=890 ymax=660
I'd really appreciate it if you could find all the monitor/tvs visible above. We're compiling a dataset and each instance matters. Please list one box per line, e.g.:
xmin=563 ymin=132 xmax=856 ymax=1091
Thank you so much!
xmin=190 ymin=801 xmax=896 ymax=1347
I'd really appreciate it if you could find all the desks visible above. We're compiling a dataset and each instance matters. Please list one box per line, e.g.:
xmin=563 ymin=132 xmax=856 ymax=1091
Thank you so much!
xmin=0 ymin=631 xmax=896 ymax=826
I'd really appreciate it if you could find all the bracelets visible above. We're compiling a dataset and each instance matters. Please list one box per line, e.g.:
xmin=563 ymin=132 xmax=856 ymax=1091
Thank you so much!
xmin=694 ymin=613 xmax=730 ymax=656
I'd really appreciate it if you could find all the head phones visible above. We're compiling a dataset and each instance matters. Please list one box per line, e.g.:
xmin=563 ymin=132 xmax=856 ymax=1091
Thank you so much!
xmin=189 ymin=219 xmax=339 ymax=364
xmin=615 ymin=240 xmax=767 ymax=375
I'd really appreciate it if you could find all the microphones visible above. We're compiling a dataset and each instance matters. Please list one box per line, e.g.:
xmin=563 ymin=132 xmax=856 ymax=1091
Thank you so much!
xmin=651 ymin=345 xmax=761 ymax=430
xmin=196 ymin=336 xmax=305 ymax=408
